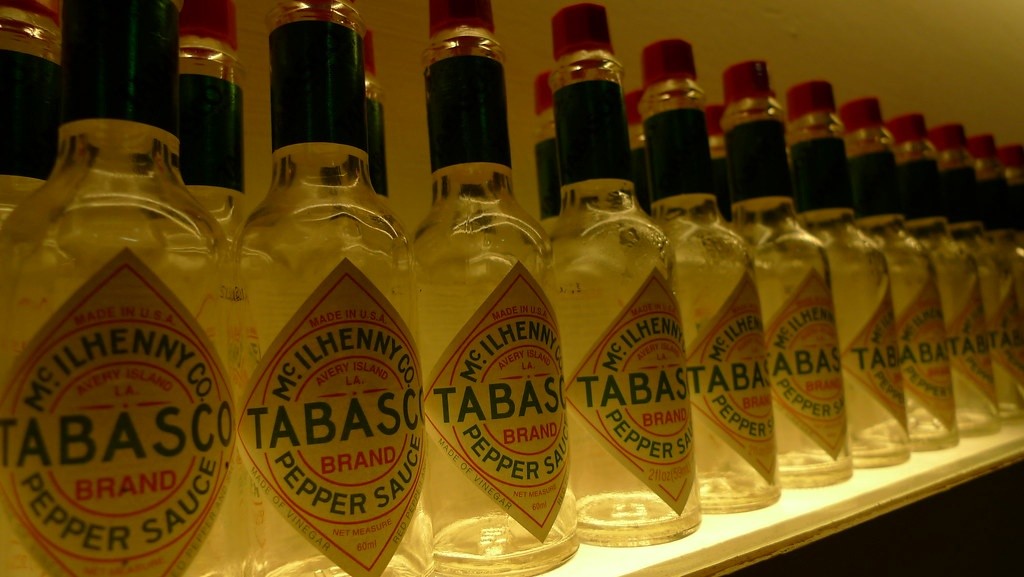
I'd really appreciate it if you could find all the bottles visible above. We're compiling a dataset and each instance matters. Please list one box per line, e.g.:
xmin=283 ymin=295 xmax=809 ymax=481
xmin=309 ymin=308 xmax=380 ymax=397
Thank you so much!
xmin=0 ymin=1 xmax=1024 ymax=577
